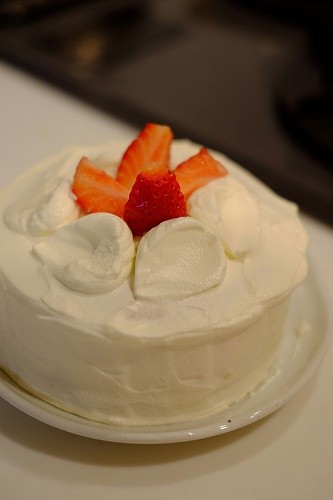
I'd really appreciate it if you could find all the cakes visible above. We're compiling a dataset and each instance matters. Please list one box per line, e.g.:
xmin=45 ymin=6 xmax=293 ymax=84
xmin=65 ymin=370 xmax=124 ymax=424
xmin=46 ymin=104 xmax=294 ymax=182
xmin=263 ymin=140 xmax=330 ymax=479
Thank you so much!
xmin=1 ymin=123 xmax=310 ymax=428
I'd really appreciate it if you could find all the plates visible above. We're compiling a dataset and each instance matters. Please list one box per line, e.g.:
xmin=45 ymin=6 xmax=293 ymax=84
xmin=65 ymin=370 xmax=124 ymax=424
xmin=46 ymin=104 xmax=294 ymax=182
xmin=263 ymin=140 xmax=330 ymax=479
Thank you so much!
xmin=1 ymin=268 xmax=326 ymax=445
